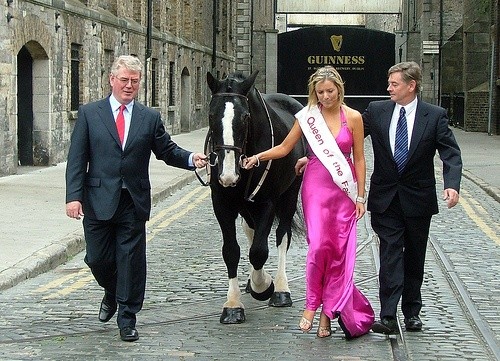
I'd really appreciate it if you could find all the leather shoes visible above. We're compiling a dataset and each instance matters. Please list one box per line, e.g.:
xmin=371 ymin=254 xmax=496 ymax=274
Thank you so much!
xmin=372 ymin=317 xmax=400 ymax=337
xmin=119 ymin=326 xmax=139 ymax=343
xmin=98 ymin=294 xmax=118 ymax=322
xmin=404 ymin=314 xmax=422 ymax=332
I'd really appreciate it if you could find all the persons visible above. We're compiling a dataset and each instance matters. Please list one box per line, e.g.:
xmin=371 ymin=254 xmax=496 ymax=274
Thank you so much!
xmin=240 ymin=65 xmax=366 ymax=338
xmin=295 ymin=60 xmax=462 ymax=334
xmin=65 ymin=55 xmax=208 ymax=341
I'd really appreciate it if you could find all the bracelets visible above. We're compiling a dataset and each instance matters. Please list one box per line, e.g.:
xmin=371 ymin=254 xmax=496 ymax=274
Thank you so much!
xmin=357 ymin=196 xmax=366 ymax=205
xmin=252 ymin=155 xmax=260 ymax=167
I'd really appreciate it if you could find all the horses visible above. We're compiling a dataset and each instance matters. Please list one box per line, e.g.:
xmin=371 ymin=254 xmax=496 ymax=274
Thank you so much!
xmin=203 ymin=67 xmax=310 ymax=323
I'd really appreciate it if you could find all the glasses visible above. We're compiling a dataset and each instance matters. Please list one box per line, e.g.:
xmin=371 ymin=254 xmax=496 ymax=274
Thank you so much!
xmin=115 ymin=77 xmax=140 ymax=85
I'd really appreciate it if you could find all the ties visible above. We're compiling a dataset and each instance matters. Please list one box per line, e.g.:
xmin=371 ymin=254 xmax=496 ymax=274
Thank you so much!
xmin=394 ymin=107 xmax=409 ymax=178
xmin=115 ymin=105 xmax=126 ymax=145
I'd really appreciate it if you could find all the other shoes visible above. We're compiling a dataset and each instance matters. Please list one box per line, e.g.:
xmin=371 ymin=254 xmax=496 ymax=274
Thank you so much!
xmin=299 ymin=316 xmax=313 ymax=332
xmin=317 ymin=324 xmax=331 ymax=338
